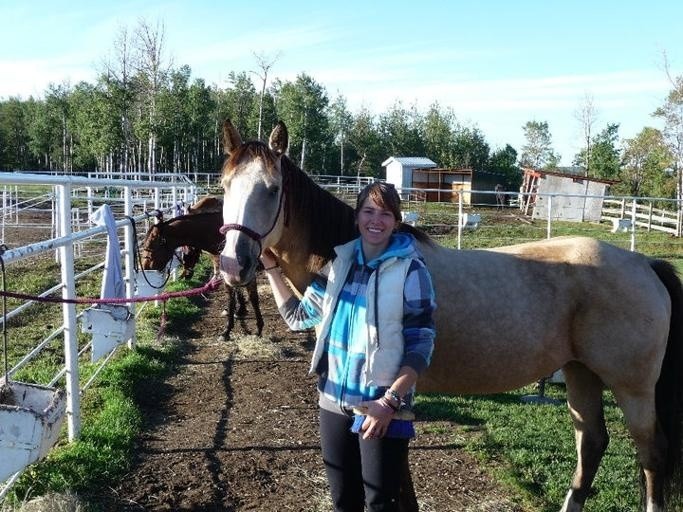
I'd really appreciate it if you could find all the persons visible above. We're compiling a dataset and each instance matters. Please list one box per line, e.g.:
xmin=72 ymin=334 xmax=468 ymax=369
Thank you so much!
xmin=257 ymin=181 xmax=437 ymax=511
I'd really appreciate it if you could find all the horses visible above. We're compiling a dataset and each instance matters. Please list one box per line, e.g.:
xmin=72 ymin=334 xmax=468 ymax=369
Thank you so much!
xmin=137 ymin=211 xmax=263 ymax=342
xmin=175 ymin=196 xmax=223 ymax=282
xmin=217 ymin=114 xmax=683 ymax=511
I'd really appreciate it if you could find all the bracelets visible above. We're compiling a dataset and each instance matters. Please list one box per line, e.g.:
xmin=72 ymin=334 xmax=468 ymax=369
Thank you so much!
xmin=385 ymin=389 xmax=406 ymax=406
xmin=262 ymin=263 xmax=279 ymax=272
xmin=381 ymin=397 xmax=398 ymax=414
